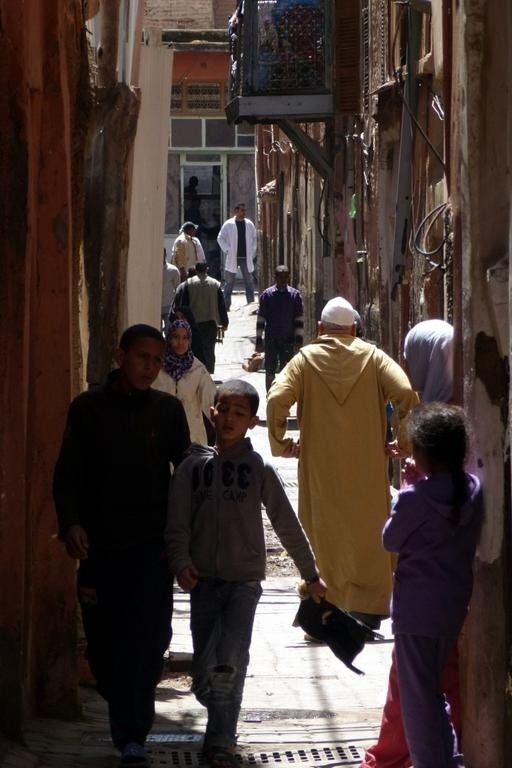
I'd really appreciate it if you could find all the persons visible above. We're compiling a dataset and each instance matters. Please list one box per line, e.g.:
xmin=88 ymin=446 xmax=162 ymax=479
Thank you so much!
xmin=51 ymin=174 xmax=490 ymax=767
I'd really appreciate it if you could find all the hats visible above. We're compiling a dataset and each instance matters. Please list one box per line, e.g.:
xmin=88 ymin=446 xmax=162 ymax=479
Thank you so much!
xmin=182 ymin=220 xmax=198 ymax=233
xmin=323 ymin=298 xmax=357 ymax=327
